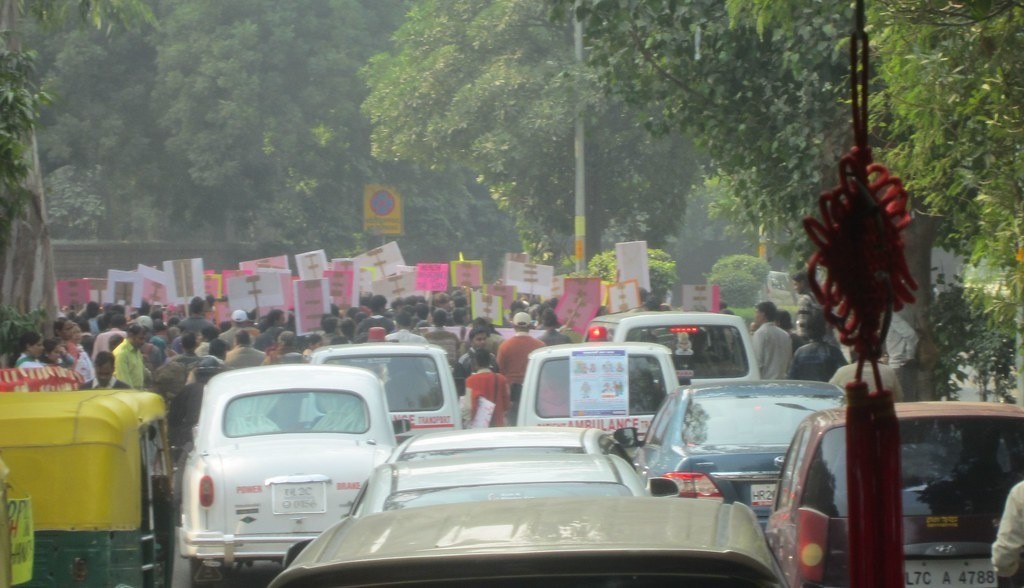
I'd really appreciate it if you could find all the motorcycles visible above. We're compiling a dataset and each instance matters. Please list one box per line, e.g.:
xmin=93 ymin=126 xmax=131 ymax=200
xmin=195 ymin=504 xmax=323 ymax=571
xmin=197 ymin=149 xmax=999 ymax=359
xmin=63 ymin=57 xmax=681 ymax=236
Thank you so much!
xmin=1 ymin=389 xmax=176 ymax=588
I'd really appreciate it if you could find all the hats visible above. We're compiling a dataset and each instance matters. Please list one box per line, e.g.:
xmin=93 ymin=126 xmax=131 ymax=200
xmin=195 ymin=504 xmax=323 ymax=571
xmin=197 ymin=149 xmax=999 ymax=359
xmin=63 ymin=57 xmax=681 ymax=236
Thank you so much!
xmin=230 ymin=310 xmax=248 ymax=322
xmin=512 ymin=312 xmax=533 ymax=327
xmin=132 ymin=316 xmax=154 ymax=330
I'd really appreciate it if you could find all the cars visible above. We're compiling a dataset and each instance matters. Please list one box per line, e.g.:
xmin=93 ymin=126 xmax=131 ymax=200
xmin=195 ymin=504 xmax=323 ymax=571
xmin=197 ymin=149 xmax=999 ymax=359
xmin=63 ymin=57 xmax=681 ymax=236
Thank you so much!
xmin=176 ymin=361 xmax=399 ymax=588
xmin=762 ymin=268 xmax=797 ymax=306
xmin=309 ymin=340 xmax=464 ymax=431
xmin=586 ymin=308 xmax=761 ymax=380
xmin=379 ymin=423 xmax=638 ymax=471
xmin=344 ymin=451 xmax=684 ymax=514
xmin=763 ymin=401 xmax=1024 ymax=588
xmin=266 ymin=495 xmax=794 ymax=588
xmin=515 ymin=339 xmax=681 ymax=446
xmin=613 ymin=378 xmax=846 ymax=525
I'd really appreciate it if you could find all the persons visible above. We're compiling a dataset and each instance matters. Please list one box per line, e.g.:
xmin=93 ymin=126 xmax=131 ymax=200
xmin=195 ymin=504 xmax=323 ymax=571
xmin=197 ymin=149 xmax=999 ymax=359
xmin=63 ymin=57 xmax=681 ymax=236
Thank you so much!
xmin=10 ymin=281 xmax=573 ymax=433
xmin=589 ymin=284 xmax=925 ymax=404
xmin=989 ymin=479 xmax=1024 ymax=588
